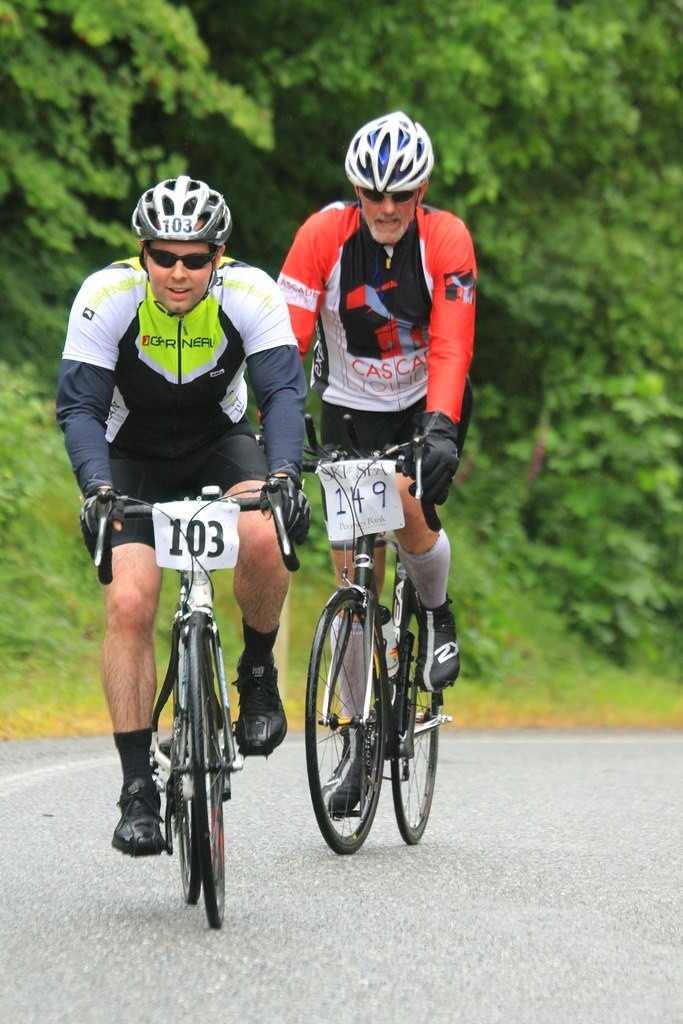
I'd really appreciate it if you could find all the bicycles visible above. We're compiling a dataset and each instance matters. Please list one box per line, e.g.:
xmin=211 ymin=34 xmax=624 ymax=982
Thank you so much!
xmin=256 ymin=412 xmax=461 ymax=858
xmin=76 ymin=475 xmax=303 ymax=931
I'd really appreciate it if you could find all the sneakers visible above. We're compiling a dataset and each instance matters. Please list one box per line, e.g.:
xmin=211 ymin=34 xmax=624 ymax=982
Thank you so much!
xmin=320 ymin=728 xmax=373 ymax=813
xmin=414 ymin=591 xmax=461 ymax=692
xmin=234 ymin=653 xmax=288 ymax=755
xmin=111 ymin=779 xmax=164 ymax=857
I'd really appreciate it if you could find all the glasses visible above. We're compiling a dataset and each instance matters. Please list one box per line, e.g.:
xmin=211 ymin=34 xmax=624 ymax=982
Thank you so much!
xmin=359 ymin=186 xmax=419 ymax=204
xmin=143 ymin=242 xmax=221 ymax=269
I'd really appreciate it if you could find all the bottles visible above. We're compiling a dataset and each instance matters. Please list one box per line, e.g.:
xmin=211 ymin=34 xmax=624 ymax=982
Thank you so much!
xmin=373 ymin=605 xmax=399 ymax=679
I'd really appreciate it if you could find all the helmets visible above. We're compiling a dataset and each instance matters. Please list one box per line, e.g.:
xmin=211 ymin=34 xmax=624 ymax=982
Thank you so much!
xmin=345 ymin=110 xmax=434 ymax=192
xmin=132 ymin=176 xmax=232 ymax=247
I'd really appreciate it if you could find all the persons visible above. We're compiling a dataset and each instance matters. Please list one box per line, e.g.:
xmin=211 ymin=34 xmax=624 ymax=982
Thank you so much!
xmin=57 ymin=175 xmax=307 ymax=855
xmin=258 ymin=112 xmax=479 ymax=813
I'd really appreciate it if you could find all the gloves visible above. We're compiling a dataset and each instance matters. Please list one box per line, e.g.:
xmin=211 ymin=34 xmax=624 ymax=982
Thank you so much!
xmin=402 ymin=411 xmax=459 ymax=506
